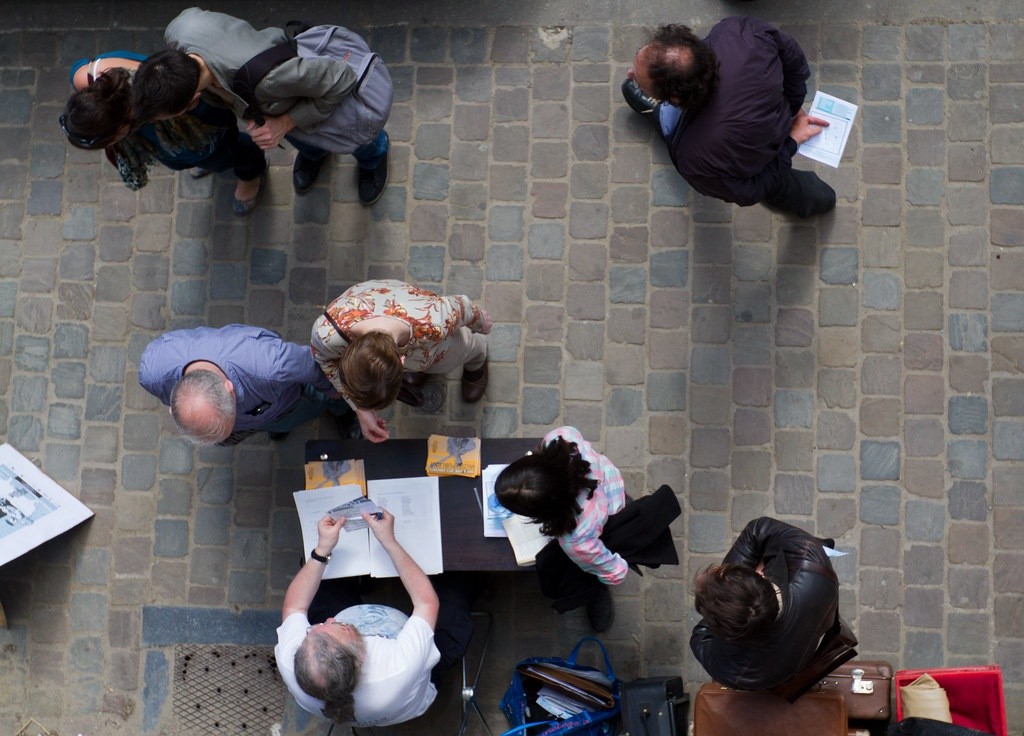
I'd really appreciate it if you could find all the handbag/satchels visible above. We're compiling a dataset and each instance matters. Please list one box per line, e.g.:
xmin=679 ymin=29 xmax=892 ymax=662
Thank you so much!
xmin=767 ymin=632 xmax=857 ymax=704
xmin=230 ymin=18 xmax=395 ymax=153
xmin=324 ymin=311 xmax=425 ymax=407
xmin=499 ymin=637 xmax=622 ymax=736
xmin=86 ymin=56 xmax=148 ymax=191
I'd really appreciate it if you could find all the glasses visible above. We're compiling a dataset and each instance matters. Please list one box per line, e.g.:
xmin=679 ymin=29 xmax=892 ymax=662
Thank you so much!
xmin=57 ymin=115 xmax=97 ymax=150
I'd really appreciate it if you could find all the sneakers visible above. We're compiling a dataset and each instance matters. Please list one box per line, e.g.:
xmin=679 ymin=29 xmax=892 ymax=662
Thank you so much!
xmin=621 ymin=78 xmax=664 ymax=125
xmin=588 ymin=586 xmax=613 ymax=632
xmin=358 ymin=131 xmax=388 ymax=205
xmin=294 ymin=151 xmax=325 ymax=193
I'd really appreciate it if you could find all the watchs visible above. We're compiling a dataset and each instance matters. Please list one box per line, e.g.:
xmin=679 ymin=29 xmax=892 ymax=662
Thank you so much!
xmin=311 ymin=549 xmax=332 ymax=564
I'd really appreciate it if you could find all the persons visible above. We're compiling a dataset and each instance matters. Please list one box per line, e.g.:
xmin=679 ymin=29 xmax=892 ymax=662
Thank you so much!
xmin=132 ymin=7 xmax=389 ymax=205
xmin=311 ymin=278 xmax=493 ymax=444
xmin=619 ymin=14 xmax=837 ymax=219
xmin=274 ymin=511 xmax=440 ymax=728
xmin=447 ymin=438 xmax=476 ymax=466
xmin=139 ymin=323 xmax=363 ymax=447
xmin=493 ymin=425 xmax=628 ymax=585
xmin=322 ymin=462 xmax=350 ymax=485
xmin=689 ymin=517 xmax=839 ymax=696
xmin=59 ymin=51 xmax=268 ymax=216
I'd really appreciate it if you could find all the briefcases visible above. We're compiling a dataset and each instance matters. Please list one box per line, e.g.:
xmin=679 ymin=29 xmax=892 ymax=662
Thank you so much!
xmin=622 ymin=676 xmax=689 ymax=736
xmin=811 ymin=660 xmax=892 ymax=720
xmin=694 ymin=678 xmax=847 ymax=736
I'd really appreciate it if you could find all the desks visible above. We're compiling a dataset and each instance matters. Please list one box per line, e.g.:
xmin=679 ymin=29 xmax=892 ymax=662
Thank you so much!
xmin=305 ymin=439 xmax=543 ymax=573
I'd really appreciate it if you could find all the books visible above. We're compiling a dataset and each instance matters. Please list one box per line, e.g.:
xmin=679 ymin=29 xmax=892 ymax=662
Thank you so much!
xmin=523 ymin=666 xmax=614 ymax=720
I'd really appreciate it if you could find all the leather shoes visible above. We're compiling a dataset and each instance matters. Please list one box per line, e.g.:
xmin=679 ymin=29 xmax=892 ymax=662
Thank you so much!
xmin=334 ymin=411 xmax=362 ymax=439
xmin=268 ymin=431 xmax=288 ymax=441
xmin=402 ymin=372 xmax=424 ymax=387
xmin=461 ymin=356 xmax=489 ymax=403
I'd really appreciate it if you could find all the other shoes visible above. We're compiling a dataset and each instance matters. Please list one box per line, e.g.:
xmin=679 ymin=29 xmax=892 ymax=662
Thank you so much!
xmin=187 ymin=166 xmax=208 ymax=178
xmin=232 ymin=170 xmax=266 ymax=215
